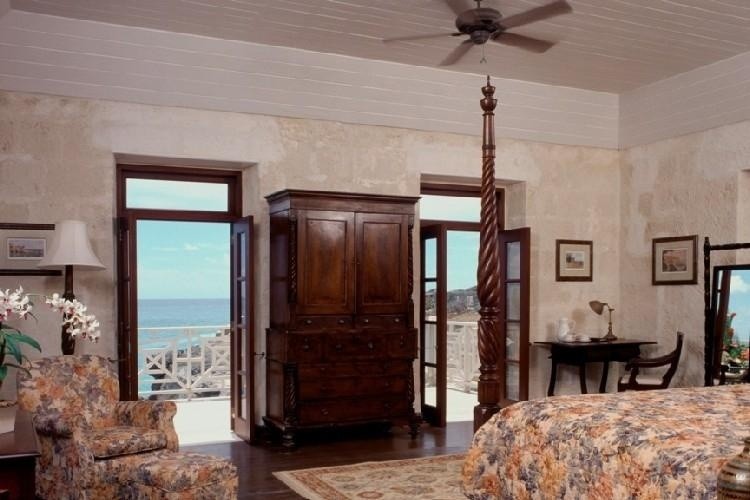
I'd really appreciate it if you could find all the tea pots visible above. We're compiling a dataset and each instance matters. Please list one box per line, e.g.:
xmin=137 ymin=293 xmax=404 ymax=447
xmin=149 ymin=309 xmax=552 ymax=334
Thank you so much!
xmin=556 ymin=317 xmax=576 ymax=343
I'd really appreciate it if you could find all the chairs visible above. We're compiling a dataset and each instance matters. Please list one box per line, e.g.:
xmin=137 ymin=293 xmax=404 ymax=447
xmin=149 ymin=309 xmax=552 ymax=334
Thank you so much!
xmin=614 ymin=331 xmax=685 ymax=393
xmin=712 ymin=361 xmax=750 ymax=386
xmin=13 ymin=354 xmax=179 ymax=500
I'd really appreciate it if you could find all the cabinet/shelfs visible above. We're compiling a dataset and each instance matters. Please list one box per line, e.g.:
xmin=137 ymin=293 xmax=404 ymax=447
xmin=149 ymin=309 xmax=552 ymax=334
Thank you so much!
xmin=260 ymin=186 xmax=428 ymax=453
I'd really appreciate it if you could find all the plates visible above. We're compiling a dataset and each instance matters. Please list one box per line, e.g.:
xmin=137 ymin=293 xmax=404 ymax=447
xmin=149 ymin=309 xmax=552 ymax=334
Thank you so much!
xmin=578 ymin=339 xmax=591 ymax=343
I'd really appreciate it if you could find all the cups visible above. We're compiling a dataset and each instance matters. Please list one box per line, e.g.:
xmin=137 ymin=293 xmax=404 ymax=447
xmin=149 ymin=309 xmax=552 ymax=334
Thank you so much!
xmin=566 ymin=333 xmax=574 ymax=343
xmin=578 ymin=336 xmax=590 ymax=340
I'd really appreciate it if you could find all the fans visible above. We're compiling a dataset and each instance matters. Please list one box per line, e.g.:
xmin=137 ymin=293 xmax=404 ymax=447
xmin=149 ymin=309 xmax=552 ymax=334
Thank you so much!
xmin=382 ymin=1 xmax=573 ymax=67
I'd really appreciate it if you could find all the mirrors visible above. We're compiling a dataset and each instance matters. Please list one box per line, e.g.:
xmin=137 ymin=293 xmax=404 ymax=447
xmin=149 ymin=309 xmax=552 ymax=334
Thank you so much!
xmin=703 ymin=235 xmax=750 ymax=386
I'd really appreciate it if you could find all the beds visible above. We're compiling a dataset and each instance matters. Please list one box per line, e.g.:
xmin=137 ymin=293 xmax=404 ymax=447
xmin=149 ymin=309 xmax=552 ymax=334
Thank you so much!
xmin=462 ymin=380 xmax=749 ymax=500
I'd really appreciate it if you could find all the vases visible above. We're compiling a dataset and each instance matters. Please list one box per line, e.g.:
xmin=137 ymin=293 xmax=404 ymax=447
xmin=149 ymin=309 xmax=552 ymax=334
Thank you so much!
xmin=713 ymin=434 xmax=749 ymax=500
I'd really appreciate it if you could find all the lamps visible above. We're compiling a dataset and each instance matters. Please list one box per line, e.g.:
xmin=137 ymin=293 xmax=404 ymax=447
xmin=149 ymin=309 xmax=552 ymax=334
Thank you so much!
xmin=588 ymin=300 xmax=619 ymax=340
xmin=36 ymin=217 xmax=106 ymax=357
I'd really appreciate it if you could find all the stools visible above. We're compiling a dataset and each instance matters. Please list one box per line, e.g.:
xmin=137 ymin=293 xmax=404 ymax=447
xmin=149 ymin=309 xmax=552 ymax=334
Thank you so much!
xmin=122 ymin=449 xmax=239 ymax=499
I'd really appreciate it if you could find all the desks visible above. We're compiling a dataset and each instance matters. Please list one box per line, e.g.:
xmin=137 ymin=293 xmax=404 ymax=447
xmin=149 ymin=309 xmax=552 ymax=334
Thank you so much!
xmin=526 ymin=336 xmax=661 ymax=395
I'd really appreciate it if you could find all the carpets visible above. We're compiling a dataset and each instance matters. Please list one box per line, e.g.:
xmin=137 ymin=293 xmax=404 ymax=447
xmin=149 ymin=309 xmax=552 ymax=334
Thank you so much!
xmin=270 ymin=447 xmax=469 ymax=500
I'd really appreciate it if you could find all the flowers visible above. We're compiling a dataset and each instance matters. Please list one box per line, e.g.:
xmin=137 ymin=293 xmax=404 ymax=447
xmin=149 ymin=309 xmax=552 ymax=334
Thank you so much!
xmin=0 ymin=285 xmax=105 ymax=401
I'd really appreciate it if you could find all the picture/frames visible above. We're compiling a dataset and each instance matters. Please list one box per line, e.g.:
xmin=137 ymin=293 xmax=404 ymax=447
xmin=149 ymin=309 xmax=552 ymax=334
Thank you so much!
xmin=0 ymin=224 xmax=62 ymax=278
xmin=555 ymin=240 xmax=593 ymax=283
xmin=650 ymin=237 xmax=697 ymax=285
